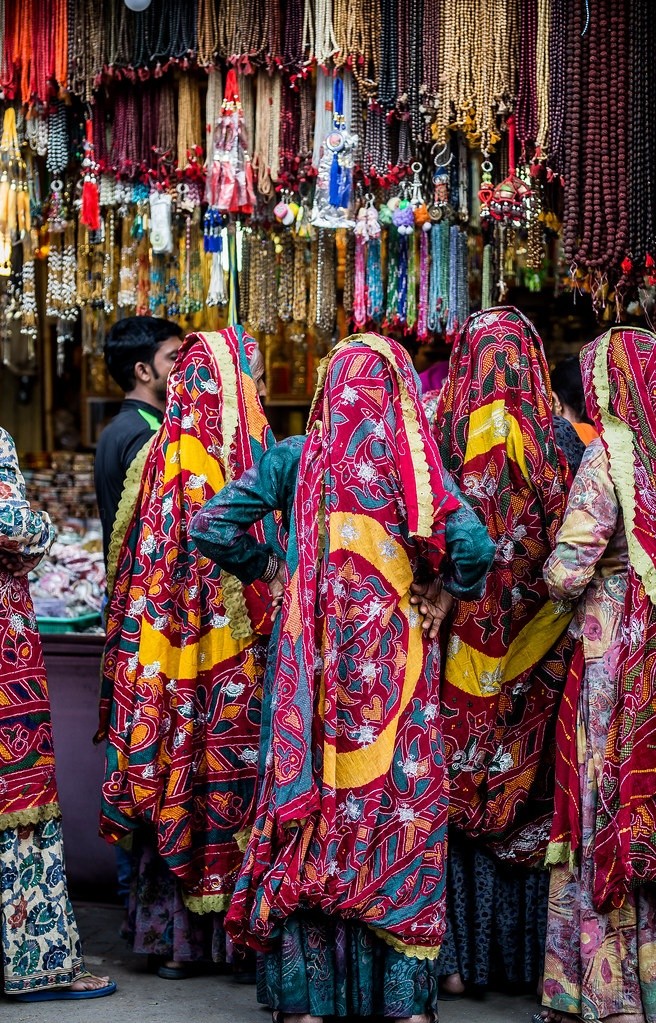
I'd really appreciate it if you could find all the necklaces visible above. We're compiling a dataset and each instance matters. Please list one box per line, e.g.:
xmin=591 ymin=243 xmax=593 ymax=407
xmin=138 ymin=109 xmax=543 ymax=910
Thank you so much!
xmin=0 ymin=0 xmax=656 ymax=378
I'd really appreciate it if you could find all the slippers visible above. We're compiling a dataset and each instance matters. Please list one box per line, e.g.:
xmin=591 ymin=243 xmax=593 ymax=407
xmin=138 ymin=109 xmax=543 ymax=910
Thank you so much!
xmin=8 ymin=978 xmax=117 ymax=1003
xmin=438 ymin=990 xmax=463 ymax=1001
xmin=531 ymin=1011 xmax=604 ymax=1023
xmin=156 ymin=966 xmax=189 ymax=979
xmin=271 ymin=1010 xmax=285 ymax=1023
xmin=118 ymin=926 xmax=134 ymax=939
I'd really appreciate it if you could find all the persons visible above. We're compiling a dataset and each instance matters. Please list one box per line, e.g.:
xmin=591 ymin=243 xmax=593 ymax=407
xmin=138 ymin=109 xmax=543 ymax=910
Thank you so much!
xmin=532 ymin=326 xmax=656 ymax=1023
xmin=0 ymin=427 xmax=117 ymax=1001
xmin=189 ymin=332 xmax=496 ymax=1023
xmin=94 ymin=316 xmax=288 ymax=980
xmin=423 ymin=305 xmax=596 ymax=999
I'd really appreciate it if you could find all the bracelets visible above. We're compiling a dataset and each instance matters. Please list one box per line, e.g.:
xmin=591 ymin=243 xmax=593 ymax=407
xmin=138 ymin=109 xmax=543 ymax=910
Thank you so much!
xmin=28 ymin=517 xmax=106 ymax=619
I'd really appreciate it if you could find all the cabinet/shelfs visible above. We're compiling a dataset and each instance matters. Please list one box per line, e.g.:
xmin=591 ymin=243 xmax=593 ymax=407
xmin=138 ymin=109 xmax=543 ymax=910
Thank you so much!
xmin=81 ymin=353 xmax=315 ymax=453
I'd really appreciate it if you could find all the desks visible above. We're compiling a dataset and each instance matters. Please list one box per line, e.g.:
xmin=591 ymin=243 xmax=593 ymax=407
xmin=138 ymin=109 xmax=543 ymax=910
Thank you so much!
xmin=37 ymin=633 xmax=131 ymax=911
